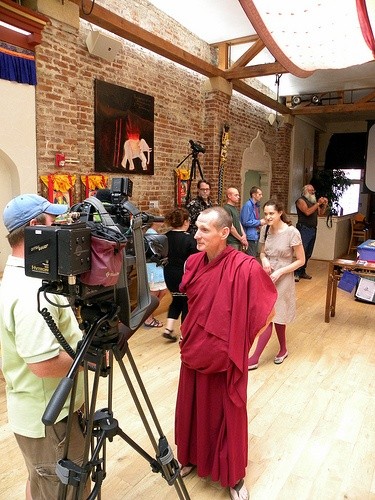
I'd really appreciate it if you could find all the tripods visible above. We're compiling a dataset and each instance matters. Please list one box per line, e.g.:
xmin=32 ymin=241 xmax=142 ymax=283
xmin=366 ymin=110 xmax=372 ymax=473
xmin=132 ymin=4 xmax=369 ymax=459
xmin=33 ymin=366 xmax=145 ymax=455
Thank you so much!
xmin=56 ymin=344 xmax=192 ymax=500
xmin=186 ymin=160 xmax=205 ymax=206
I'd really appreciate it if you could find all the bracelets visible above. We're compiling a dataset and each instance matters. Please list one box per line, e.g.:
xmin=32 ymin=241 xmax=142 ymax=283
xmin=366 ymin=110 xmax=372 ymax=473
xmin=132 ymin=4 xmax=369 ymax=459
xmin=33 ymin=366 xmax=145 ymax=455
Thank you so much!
xmin=316 ymin=203 xmax=319 ymax=205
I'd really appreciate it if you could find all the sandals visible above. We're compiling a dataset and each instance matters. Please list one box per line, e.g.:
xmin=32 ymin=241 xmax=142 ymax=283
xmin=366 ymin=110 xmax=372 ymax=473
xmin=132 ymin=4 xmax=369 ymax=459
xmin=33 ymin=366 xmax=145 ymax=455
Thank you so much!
xmin=164 ymin=329 xmax=177 ymax=340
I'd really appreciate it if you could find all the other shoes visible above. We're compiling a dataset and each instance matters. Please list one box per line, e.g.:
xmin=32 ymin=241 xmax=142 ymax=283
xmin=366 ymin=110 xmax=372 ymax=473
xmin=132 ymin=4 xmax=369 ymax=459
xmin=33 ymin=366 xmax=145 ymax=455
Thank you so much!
xmin=275 ymin=351 xmax=287 ymax=363
xmin=300 ymin=273 xmax=311 ymax=279
xmin=248 ymin=363 xmax=258 ymax=370
xmin=295 ymin=276 xmax=300 ymax=281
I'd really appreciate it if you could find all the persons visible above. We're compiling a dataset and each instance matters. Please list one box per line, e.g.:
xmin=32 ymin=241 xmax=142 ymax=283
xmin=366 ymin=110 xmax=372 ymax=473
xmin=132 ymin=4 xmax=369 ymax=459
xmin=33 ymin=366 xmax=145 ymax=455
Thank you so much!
xmin=248 ymin=199 xmax=306 ymax=370
xmin=162 ymin=208 xmax=202 ymax=341
xmin=175 ymin=205 xmax=277 ymax=500
xmin=240 ymin=186 xmax=266 ymax=258
xmin=0 ymin=194 xmax=109 ymax=500
xmin=143 ymin=209 xmax=167 ymax=328
xmin=294 ymin=184 xmax=328 ymax=282
xmin=185 ymin=179 xmax=214 ymax=234
xmin=222 ymin=187 xmax=249 ymax=252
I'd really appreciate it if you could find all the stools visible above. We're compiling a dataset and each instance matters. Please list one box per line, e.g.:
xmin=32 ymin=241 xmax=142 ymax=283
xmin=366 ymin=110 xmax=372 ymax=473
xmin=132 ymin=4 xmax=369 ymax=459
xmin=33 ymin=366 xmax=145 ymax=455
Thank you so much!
xmin=347 ymin=218 xmax=371 ymax=256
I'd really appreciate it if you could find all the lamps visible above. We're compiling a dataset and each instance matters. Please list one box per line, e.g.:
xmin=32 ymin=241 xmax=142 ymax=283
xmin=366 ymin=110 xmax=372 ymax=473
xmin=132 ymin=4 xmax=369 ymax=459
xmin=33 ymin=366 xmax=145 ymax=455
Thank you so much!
xmin=292 ymin=94 xmax=319 ymax=104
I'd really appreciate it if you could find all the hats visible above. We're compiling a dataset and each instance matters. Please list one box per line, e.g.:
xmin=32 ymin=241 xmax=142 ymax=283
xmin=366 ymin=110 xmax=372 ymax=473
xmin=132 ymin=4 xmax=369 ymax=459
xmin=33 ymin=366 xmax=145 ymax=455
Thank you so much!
xmin=3 ymin=194 xmax=68 ymax=232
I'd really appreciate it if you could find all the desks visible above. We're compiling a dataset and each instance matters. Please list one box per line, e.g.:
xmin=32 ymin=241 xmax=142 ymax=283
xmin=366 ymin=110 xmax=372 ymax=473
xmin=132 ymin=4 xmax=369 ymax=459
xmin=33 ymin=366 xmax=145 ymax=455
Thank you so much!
xmin=357 ymin=239 xmax=375 ymax=261
xmin=325 ymin=258 xmax=375 ymax=323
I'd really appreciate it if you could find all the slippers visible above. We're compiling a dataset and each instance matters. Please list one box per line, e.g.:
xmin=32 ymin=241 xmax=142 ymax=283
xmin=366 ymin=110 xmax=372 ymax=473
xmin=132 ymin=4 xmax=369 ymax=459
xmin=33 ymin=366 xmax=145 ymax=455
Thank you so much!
xmin=146 ymin=319 xmax=163 ymax=327
xmin=181 ymin=464 xmax=195 ymax=478
xmin=229 ymin=478 xmax=249 ymax=500
